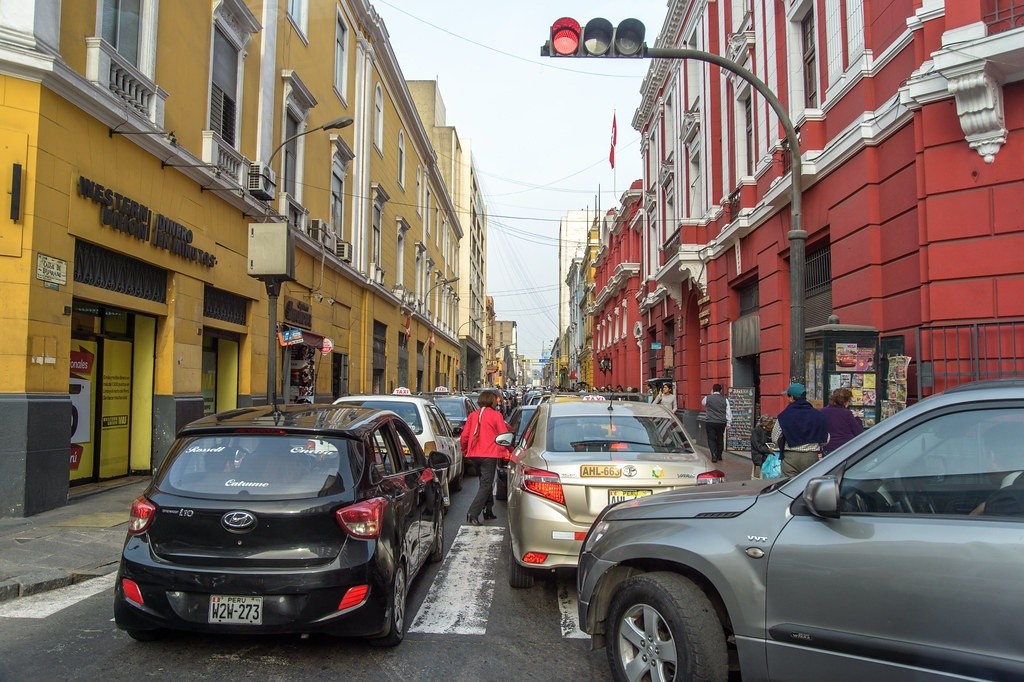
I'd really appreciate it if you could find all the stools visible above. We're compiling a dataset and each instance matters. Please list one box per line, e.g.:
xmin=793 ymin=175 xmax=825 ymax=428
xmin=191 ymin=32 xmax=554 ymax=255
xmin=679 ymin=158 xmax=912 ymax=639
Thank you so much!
xmin=750 ymin=463 xmax=763 ymax=480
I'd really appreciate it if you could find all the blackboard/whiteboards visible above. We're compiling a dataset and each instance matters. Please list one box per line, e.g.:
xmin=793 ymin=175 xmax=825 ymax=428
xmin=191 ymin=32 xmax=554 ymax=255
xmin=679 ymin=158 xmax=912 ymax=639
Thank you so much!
xmin=725 ymin=386 xmax=755 ymax=451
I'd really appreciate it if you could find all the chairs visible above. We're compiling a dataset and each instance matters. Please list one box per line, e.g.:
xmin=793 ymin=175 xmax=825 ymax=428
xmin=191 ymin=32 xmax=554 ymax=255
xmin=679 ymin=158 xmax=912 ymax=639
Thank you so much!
xmin=263 ymin=453 xmax=309 ymax=483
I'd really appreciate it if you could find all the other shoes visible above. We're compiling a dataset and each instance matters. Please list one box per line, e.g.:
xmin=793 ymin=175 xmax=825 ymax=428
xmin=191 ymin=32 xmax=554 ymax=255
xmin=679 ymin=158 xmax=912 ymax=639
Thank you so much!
xmin=712 ymin=454 xmax=717 ymax=462
xmin=717 ymin=453 xmax=723 ymax=461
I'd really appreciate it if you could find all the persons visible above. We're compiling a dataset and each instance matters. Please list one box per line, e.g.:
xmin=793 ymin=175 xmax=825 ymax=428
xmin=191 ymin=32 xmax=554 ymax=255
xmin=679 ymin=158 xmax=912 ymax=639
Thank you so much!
xmin=453 ymin=387 xmax=458 ymax=392
xmin=969 ymin=422 xmax=1024 ymax=518
xmin=629 ymin=388 xmax=639 ymax=402
xmin=820 ymin=388 xmax=863 ymax=454
xmin=550 ymin=385 xmax=575 ymax=392
xmin=204 ymin=444 xmax=236 ymax=473
xmin=460 ymin=390 xmax=516 ymax=525
xmin=772 ymin=383 xmax=830 ymax=480
xmin=653 ymin=383 xmax=678 ymax=414
xmin=701 ymin=384 xmax=733 ymax=463
xmin=641 ymin=383 xmax=653 ymax=404
xmin=751 ymin=414 xmax=774 ymax=466
xmin=592 ymin=384 xmax=628 ymax=401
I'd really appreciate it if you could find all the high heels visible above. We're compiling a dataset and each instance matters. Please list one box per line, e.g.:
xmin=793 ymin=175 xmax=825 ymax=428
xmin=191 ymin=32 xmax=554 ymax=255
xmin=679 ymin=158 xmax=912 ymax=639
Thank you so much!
xmin=467 ymin=512 xmax=483 ymax=526
xmin=482 ymin=508 xmax=497 ymax=520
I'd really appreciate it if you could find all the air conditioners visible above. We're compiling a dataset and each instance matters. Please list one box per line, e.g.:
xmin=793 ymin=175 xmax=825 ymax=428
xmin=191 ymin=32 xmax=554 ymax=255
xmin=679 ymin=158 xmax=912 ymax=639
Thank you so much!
xmin=247 ymin=161 xmax=276 ymax=200
xmin=310 ymin=218 xmax=332 ymax=245
xmin=336 ymin=241 xmax=352 ymax=262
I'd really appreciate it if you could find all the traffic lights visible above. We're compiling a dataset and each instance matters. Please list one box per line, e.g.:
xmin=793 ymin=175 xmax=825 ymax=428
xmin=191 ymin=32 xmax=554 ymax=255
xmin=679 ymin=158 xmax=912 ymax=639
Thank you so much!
xmin=548 ymin=17 xmax=646 ymax=57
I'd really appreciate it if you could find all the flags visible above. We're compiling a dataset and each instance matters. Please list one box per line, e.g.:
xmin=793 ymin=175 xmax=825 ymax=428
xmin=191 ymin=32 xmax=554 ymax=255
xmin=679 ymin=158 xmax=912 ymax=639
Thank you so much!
xmin=406 ymin=315 xmax=411 ymax=340
xmin=429 ymin=329 xmax=436 ymax=349
xmin=609 ymin=113 xmax=616 ymax=169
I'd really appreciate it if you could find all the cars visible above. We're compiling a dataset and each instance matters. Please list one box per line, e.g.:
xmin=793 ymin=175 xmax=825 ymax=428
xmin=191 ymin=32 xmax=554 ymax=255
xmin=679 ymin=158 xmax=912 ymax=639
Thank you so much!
xmin=330 ymin=387 xmax=464 ymax=517
xmin=112 ymin=403 xmax=452 ymax=651
xmin=493 ymin=399 xmax=726 ymax=590
xmin=414 ymin=384 xmax=649 ymax=500
xmin=576 ymin=377 xmax=1024 ymax=682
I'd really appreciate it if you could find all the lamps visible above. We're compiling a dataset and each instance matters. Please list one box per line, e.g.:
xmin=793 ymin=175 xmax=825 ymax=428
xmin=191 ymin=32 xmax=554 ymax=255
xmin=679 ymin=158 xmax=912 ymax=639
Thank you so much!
xmin=457 ymin=318 xmax=480 ymax=333
xmin=109 ymin=127 xmax=178 ymax=148
xmin=599 ymin=357 xmax=612 ymax=376
xmin=425 ymin=277 xmax=460 ymax=299
xmin=268 ymin=116 xmax=353 ymax=167
xmin=242 ymin=212 xmax=289 ymax=223
xmin=291 ymin=290 xmax=323 ymax=302
xmin=201 ymin=185 xmax=245 ymax=198
xmin=162 ymin=160 xmax=222 ymax=178
xmin=303 ymin=295 xmax=335 ymax=306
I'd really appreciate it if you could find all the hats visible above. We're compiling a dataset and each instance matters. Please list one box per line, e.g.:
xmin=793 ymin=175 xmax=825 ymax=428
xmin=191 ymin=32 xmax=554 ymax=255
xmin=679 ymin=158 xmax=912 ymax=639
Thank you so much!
xmin=781 ymin=383 xmax=807 ymax=399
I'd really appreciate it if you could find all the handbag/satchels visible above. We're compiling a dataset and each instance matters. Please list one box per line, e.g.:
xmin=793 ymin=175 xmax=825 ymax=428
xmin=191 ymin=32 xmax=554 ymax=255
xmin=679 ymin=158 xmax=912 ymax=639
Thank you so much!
xmin=761 ymin=452 xmax=783 ymax=480
